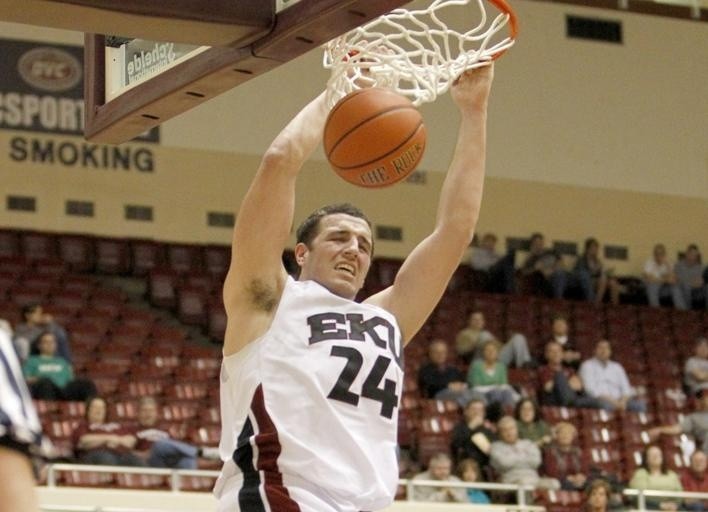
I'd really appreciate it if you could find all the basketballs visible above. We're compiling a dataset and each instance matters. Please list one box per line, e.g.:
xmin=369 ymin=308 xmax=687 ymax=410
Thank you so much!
xmin=322 ymin=87 xmax=428 ymax=187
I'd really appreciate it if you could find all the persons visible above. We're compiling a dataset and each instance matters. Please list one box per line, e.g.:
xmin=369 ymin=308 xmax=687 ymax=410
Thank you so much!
xmin=471 ymin=233 xmax=708 ymax=310
xmin=210 ymin=39 xmax=495 ymax=512
xmin=0 ymin=301 xmax=222 ymax=512
xmin=398 ymin=311 xmax=708 ymax=510
